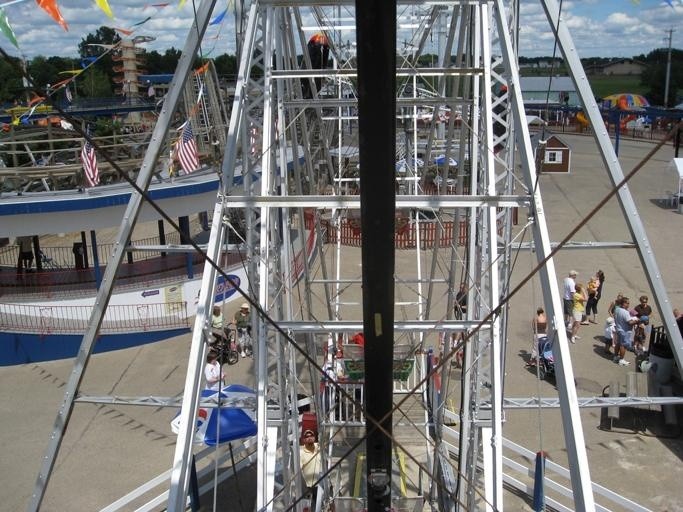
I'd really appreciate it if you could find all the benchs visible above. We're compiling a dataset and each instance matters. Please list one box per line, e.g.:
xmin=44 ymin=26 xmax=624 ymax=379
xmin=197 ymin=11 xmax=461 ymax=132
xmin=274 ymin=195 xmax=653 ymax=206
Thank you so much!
xmin=602 ymin=380 xmax=679 ymax=437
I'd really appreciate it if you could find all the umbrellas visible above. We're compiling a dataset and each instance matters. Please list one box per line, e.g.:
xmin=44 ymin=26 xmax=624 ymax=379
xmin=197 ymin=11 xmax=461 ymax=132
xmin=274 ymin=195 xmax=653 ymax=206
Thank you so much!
xmin=168 ymin=383 xmax=280 ymax=512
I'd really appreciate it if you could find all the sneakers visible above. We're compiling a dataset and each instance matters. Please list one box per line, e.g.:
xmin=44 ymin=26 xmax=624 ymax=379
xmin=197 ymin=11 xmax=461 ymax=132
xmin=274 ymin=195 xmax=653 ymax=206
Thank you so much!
xmin=565 ymin=320 xmax=598 ymax=344
xmin=241 ymin=349 xmax=251 ymax=358
xmin=614 ymin=356 xmax=632 ymax=365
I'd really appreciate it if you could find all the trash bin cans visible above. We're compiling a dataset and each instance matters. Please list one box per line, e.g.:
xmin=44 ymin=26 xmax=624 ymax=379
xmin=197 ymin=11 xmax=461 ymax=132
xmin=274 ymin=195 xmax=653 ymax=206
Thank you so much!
xmin=299 ymin=412 xmax=318 ymax=446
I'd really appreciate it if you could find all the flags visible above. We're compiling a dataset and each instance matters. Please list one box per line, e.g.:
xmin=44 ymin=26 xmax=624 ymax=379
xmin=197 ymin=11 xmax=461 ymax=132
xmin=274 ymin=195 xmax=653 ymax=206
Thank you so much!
xmin=81 ymin=140 xmax=100 ymax=187
xmin=175 ymin=120 xmax=202 ymax=174
xmin=250 ymin=111 xmax=259 ymax=154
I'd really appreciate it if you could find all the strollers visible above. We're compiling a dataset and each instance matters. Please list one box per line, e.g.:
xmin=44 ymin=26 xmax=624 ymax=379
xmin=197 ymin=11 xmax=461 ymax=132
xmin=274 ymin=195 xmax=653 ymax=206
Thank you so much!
xmin=206 ymin=322 xmax=239 ymax=368
xmin=537 ymin=336 xmax=556 ymax=380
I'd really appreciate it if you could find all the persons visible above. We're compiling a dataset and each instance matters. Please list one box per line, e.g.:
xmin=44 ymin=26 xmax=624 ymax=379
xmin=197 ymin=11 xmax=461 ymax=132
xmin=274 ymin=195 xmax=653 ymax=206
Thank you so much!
xmin=16 ymin=236 xmax=35 ymax=272
xmin=300 ymin=429 xmax=322 ymax=487
xmin=232 ymin=302 xmax=251 ymax=358
xmin=77 ymin=243 xmax=87 ymax=272
xmin=562 ymin=269 xmax=683 ymax=367
xmin=452 ymin=282 xmax=467 ymax=348
xmin=212 ymin=305 xmax=226 ymax=330
xmin=204 ymin=352 xmax=227 ymax=390
xmin=526 ymin=306 xmax=548 ymax=366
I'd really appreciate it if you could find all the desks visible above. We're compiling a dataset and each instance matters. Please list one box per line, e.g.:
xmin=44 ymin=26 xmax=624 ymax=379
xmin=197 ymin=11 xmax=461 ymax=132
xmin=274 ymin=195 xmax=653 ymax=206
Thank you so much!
xmin=623 ymin=371 xmax=662 ymax=435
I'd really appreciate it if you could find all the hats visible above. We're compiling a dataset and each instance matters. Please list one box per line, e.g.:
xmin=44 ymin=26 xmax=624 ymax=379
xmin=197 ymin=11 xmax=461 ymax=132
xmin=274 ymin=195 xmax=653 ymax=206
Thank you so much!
xmin=630 ymin=309 xmax=638 ymax=315
xmin=241 ymin=303 xmax=249 ymax=308
xmin=640 ymin=315 xmax=649 ymax=321
xmin=606 ymin=317 xmax=613 ymax=325
xmin=570 ymin=270 xmax=579 ymax=275
